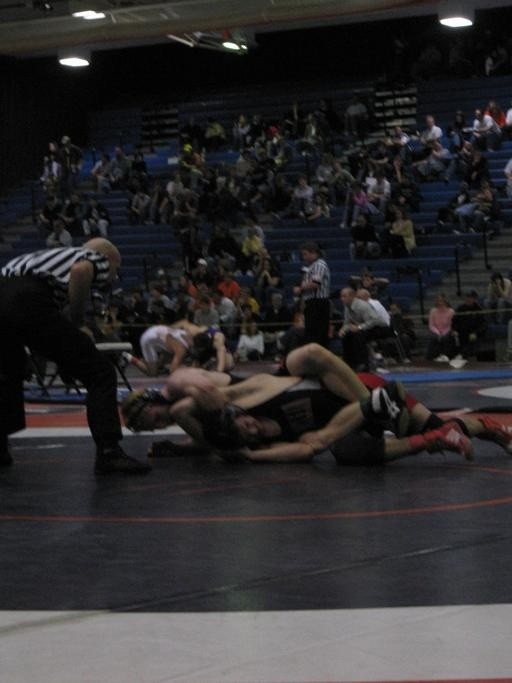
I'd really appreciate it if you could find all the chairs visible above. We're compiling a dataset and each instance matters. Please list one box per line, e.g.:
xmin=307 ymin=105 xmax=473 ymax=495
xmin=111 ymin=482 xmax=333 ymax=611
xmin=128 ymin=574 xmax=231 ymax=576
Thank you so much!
xmin=0 ymin=113 xmax=512 ymax=392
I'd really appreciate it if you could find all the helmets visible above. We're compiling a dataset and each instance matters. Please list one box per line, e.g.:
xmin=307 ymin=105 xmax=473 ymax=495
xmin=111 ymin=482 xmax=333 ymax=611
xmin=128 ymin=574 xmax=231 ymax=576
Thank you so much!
xmin=207 ymin=401 xmax=248 ymax=453
xmin=120 ymin=387 xmax=163 ymax=433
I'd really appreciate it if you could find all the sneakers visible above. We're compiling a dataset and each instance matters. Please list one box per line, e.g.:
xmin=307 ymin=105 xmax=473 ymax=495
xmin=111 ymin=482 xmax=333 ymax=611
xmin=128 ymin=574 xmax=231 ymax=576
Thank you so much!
xmin=40 ymin=122 xmax=509 ymax=375
xmin=370 ymin=378 xmax=413 ymax=438
xmin=475 ymin=413 xmax=512 ymax=455
xmin=91 ymin=445 xmax=152 ymax=477
xmin=424 ymin=417 xmax=476 ymax=463
xmin=118 ymin=351 xmax=131 ymax=376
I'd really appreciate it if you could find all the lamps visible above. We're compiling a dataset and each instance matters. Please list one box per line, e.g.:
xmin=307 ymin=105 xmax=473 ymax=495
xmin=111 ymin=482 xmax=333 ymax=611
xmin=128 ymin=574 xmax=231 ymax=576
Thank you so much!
xmin=435 ymin=1 xmax=476 ymax=28
xmin=58 ymin=43 xmax=92 ymax=67
